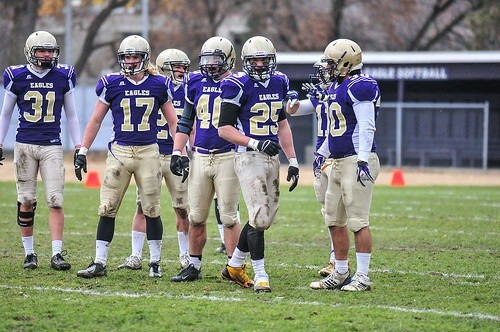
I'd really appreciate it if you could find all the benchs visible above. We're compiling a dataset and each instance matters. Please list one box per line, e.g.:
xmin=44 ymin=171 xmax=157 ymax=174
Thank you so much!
xmin=387 ymin=149 xmax=460 ymax=167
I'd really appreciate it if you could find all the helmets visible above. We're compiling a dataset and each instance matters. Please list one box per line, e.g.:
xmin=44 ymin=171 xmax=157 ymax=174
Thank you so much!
xmin=242 ymin=36 xmax=276 ymax=82
xmin=198 ymin=37 xmax=236 ymax=78
xmin=321 ymin=39 xmax=363 ymax=82
xmin=156 ymin=48 xmax=192 ymax=86
xmin=115 ymin=35 xmax=150 ymax=75
xmin=310 ymin=57 xmax=331 ymax=90
xmin=23 ymin=31 xmax=59 ymax=69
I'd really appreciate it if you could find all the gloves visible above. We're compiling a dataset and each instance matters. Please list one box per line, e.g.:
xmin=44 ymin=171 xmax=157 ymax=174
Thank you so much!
xmin=170 ymin=155 xmax=182 ymax=176
xmin=312 ymin=155 xmax=325 ymax=176
xmin=258 ymin=139 xmax=282 ymax=158
xmin=355 ymin=162 xmax=373 ymax=186
xmin=287 ymin=157 xmax=300 ymax=191
xmin=74 ymin=148 xmax=88 ymax=181
xmin=0 ymin=148 xmax=6 ymax=166
xmin=181 ymin=156 xmax=189 ymax=183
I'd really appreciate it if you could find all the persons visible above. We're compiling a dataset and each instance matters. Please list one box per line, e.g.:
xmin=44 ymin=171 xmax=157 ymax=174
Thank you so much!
xmin=287 ymin=56 xmax=350 ymax=276
xmin=0 ymin=31 xmax=82 ymax=271
xmin=217 ymin=35 xmax=299 ymax=293
xmin=301 ymin=39 xmax=381 ymax=293
xmin=169 ymin=37 xmax=246 ymax=282
xmin=74 ymin=34 xmax=189 ymax=279
xmin=118 ymin=48 xmax=240 ymax=270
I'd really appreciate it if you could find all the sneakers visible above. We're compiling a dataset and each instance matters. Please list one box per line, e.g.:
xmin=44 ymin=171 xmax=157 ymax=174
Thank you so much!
xmin=51 ymin=249 xmax=71 ymax=270
xmin=341 ymin=277 xmax=372 ymax=290
xmin=119 ymin=255 xmax=142 ymax=270
xmin=309 ymin=269 xmax=352 ymax=289
xmin=170 ymin=242 xmax=225 ymax=282
xmin=77 ymin=257 xmax=108 ymax=278
xmin=319 ymin=261 xmax=334 ymax=276
xmin=221 ymin=263 xmax=255 ymax=288
xmin=24 ymin=253 xmax=38 ymax=269
xmin=254 ymin=272 xmax=272 ymax=291
xmin=148 ymin=259 xmax=162 ymax=277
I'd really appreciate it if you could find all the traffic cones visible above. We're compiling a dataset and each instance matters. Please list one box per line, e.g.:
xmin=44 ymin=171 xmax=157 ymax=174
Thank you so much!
xmin=84 ymin=171 xmax=101 ymax=188
xmin=392 ymin=170 xmax=406 ymax=185
